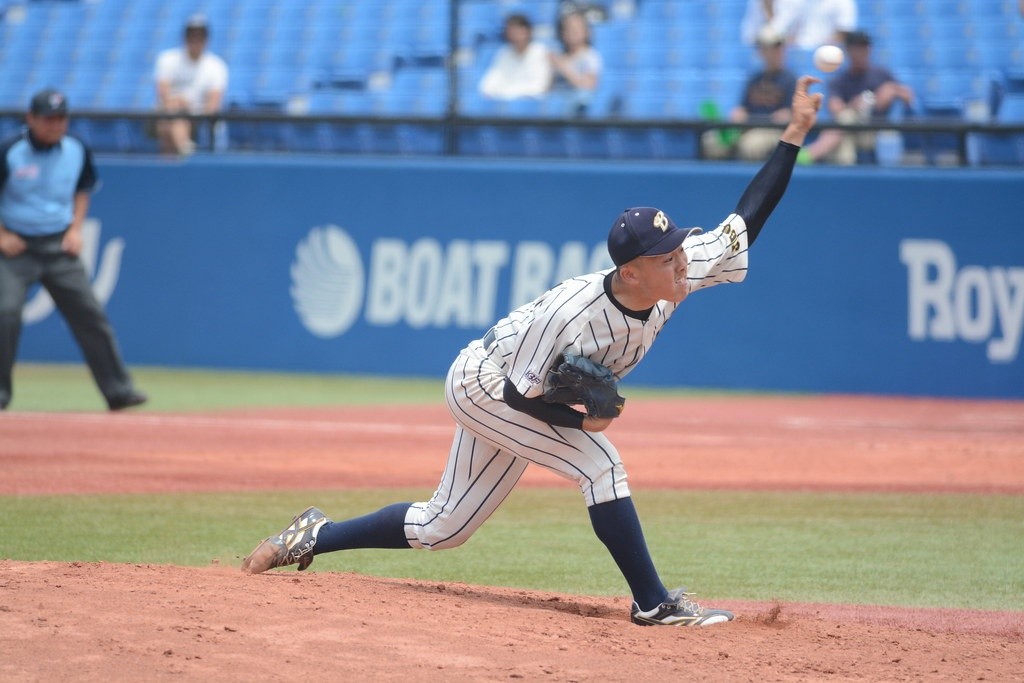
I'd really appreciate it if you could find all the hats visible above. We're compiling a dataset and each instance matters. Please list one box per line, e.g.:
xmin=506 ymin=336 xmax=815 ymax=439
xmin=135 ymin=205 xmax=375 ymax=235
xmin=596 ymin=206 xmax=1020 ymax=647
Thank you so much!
xmin=607 ymin=207 xmax=702 ymax=268
xmin=31 ymin=88 xmax=66 ymax=118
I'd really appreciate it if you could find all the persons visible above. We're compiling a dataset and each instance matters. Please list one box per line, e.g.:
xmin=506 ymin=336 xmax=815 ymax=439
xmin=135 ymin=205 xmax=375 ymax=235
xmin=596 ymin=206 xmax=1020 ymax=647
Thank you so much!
xmin=154 ymin=14 xmax=229 ymax=154
xmin=796 ymin=30 xmax=898 ymax=164
xmin=0 ymin=87 xmax=145 ymax=412
xmin=742 ymin=0 xmax=857 ymax=73
xmin=478 ymin=12 xmax=554 ymax=100
xmin=548 ymin=14 xmax=602 ymax=88
xmin=702 ymin=33 xmax=798 ymax=160
xmin=240 ymin=75 xmax=825 ymax=627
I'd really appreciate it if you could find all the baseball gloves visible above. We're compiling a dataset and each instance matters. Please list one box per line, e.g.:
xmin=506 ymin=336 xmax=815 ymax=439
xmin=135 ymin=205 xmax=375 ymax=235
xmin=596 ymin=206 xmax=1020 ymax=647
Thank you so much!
xmin=540 ymin=353 xmax=627 ymax=420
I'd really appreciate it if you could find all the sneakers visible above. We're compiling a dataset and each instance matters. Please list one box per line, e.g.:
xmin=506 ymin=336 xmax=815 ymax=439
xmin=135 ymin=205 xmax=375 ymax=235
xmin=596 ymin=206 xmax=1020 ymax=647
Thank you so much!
xmin=631 ymin=588 xmax=735 ymax=627
xmin=241 ymin=506 xmax=333 ymax=573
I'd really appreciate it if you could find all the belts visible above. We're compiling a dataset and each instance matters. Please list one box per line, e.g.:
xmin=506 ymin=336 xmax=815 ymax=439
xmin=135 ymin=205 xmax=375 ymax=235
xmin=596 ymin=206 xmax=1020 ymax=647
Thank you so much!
xmin=484 ymin=327 xmax=495 ymax=350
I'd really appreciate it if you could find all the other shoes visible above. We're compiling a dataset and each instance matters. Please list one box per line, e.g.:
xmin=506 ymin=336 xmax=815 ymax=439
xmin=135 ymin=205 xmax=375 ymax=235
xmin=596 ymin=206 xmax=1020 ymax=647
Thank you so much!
xmin=109 ymin=388 xmax=145 ymax=410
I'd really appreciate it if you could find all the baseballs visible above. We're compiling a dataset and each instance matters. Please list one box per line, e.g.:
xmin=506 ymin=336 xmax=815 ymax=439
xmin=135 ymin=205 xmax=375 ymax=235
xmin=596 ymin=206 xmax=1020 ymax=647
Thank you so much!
xmin=812 ymin=44 xmax=845 ymax=73
